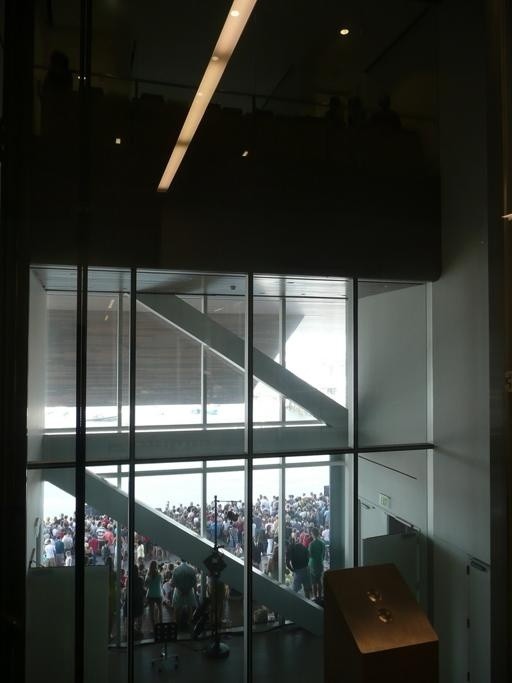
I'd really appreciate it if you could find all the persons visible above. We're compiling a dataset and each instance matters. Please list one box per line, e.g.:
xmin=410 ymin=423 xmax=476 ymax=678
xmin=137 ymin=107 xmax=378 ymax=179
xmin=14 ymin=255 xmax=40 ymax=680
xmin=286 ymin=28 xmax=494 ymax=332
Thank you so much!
xmin=43 ymin=492 xmax=330 ymax=644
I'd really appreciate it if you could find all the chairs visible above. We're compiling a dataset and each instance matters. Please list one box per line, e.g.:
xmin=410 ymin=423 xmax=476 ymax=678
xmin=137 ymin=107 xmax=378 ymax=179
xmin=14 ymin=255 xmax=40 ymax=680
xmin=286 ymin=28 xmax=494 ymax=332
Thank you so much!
xmin=152 ymin=622 xmax=179 ymax=673
